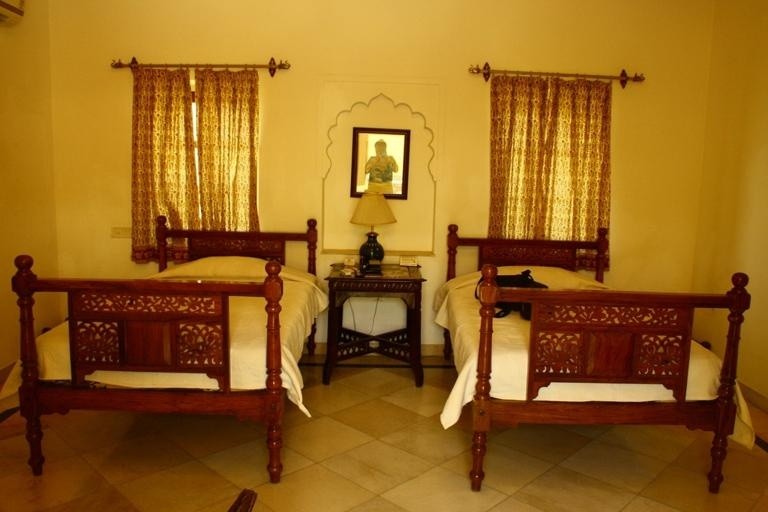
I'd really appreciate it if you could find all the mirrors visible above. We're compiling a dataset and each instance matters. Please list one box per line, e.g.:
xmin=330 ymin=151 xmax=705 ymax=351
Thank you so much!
xmin=349 ymin=126 xmax=411 ymax=200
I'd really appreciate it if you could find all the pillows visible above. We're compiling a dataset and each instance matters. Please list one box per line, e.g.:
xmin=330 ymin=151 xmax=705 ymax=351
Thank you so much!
xmin=146 ymin=256 xmax=318 ymax=288
xmin=455 ymin=265 xmax=611 ymax=289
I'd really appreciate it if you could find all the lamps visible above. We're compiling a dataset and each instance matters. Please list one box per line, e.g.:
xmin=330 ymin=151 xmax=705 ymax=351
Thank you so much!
xmin=348 ymin=193 xmax=397 ymax=273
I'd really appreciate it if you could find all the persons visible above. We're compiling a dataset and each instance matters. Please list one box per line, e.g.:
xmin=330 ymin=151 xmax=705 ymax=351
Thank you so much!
xmin=365 ymin=139 xmax=399 ymax=194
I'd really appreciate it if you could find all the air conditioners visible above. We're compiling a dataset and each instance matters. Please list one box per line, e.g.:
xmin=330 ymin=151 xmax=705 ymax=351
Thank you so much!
xmin=0 ymin=0 xmax=25 ymax=28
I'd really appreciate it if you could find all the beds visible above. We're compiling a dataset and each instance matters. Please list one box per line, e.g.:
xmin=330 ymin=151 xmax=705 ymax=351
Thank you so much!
xmin=432 ymin=224 xmax=756 ymax=493
xmin=0 ymin=215 xmax=330 ymax=476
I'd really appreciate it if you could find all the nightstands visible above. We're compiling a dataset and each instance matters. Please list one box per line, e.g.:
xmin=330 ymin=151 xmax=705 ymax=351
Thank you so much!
xmin=321 ymin=263 xmax=427 ymax=388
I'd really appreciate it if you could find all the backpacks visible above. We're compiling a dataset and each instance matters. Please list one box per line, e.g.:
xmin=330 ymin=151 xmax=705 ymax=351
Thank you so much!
xmin=474 ymin=269 xmax=548 ymax=320
